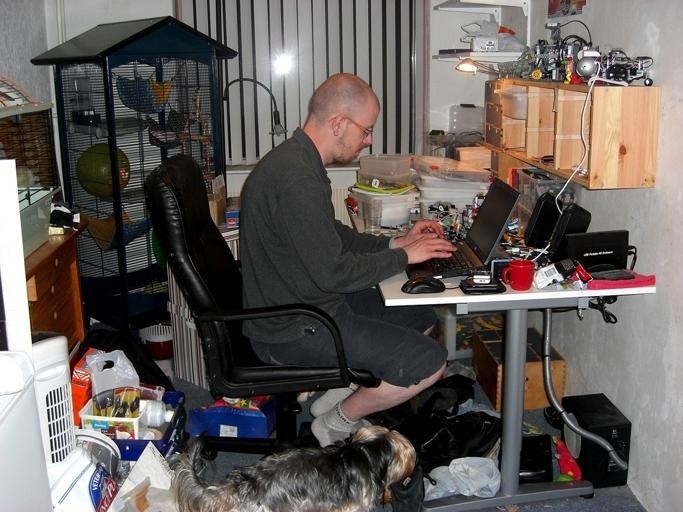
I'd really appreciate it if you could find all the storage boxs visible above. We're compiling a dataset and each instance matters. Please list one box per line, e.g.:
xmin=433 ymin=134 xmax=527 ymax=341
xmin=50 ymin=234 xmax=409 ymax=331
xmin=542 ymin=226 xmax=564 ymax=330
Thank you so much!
xmin=493 ymin=87 xmax=528 ymax=120
xmin=515 ymin=202 xmax=537 ymax=239
xmin=470 ymin=326 xmax=564 ymax=409
xmin=514 ymin=166 xmax=581 ymax=213
xmin=76 ymin=386 xmax=164 ymax=439
xmin=99 ymin=386 xmax=190 ymax=463
xmin=347 ymin=145 xmax=491 ymax=228
xmin=206 ymin=172 xmax=227 ymax=225
xmin=188 ymin=397 xmax=277 ymax=439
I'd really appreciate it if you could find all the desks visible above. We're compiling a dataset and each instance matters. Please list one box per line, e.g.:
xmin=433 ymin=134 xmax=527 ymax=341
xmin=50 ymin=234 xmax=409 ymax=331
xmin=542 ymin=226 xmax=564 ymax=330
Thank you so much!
xmin=341 ymin=187 xmax=658 ymax=511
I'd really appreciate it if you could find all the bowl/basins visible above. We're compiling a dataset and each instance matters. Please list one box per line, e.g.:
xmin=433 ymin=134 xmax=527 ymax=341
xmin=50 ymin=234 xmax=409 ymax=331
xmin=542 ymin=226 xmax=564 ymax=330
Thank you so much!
xmin=77 ymin=113 xmax=102 ymax=128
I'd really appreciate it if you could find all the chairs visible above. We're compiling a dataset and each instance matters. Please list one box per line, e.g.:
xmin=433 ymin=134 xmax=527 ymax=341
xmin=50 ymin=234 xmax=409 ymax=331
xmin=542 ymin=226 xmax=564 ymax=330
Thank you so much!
xmin=138 ymin=153 xmax=382 ymax=463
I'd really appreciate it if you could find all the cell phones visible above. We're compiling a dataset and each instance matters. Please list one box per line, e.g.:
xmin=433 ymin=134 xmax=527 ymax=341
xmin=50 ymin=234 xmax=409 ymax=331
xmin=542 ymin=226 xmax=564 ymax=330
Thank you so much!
xmin=472 ymin=270 xmax=492 ymax=285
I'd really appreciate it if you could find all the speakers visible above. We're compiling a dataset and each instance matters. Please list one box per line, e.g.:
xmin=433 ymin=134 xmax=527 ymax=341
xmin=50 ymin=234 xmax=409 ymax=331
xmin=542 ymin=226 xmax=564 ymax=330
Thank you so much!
xmin=548 ymin=202 xmax=592 ymax=265
xmin=561 ymin=393 xmax=631 ymax=488
xmin=401 ymin=278 xmax=445 ymax=294
xmin=523 ymin=193 xmax=562 ymax=250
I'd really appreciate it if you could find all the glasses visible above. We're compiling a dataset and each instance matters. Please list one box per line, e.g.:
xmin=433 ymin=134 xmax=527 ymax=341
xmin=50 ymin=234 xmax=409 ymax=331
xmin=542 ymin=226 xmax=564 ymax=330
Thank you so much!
xmin=327 ymin=110 xmax=374 ymax=138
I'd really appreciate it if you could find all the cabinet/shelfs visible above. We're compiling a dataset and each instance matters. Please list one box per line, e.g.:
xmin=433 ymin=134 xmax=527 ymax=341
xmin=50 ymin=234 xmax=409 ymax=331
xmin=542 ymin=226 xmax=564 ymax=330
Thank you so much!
xmin=481 ymin=76 xmax=659 ymax=195
xmin=24 ymin=218 xmax=90 ymax=363
xmin=426 ymin=0 xmax=531 ymax=65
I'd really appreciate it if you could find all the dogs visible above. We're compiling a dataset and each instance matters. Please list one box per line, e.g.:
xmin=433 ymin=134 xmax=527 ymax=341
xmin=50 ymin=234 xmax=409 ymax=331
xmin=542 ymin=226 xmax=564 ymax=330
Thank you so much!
xmin=169 ymin=423 xmax=419 ymax=512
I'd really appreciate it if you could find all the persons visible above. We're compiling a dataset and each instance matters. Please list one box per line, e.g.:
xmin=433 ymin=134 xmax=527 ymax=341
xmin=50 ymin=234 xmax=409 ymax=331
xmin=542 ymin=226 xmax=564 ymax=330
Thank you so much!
xmin=238 ymin=73 xmax=459 ymax=451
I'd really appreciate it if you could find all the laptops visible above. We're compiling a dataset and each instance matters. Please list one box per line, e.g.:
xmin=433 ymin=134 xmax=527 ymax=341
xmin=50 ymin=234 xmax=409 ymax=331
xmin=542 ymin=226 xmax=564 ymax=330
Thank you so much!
xmin=405 ymin=177 xmax=520 ymax=281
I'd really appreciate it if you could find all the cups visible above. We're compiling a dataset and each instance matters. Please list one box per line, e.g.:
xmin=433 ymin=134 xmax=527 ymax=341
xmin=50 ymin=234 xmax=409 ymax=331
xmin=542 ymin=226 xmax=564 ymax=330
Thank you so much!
xmin=362 ymin=198 xmax=382 ymax=236
xmin=502 ymin=259 xmax=535 ymax=291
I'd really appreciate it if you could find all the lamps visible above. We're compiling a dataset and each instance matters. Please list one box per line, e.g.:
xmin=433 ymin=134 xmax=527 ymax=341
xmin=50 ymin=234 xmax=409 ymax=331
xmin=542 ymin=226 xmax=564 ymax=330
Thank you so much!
xmin=454 ymin=58 xmax=506 ymax=77
xmin=220 ymin=78 xmax=286 ymax=138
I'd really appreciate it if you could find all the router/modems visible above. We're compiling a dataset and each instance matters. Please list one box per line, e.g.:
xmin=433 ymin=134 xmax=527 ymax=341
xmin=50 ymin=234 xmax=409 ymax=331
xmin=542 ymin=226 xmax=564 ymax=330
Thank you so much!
xmin=566 ymin=229 xmax=630 ymax=273
